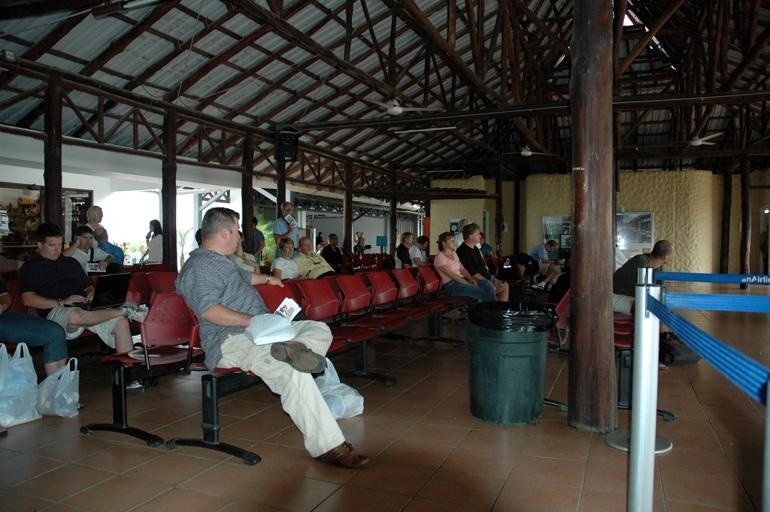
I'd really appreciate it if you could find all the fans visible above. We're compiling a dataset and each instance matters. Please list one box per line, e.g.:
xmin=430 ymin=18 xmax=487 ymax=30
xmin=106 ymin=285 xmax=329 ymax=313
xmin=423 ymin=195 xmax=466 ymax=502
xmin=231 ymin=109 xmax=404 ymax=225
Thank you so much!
xmin=677 ymin=132 xmax=725 ymax=148
xmin=369 ymin=90 xmax=448 ymax=122
xmin=502 ymin=140 xmax=559 ymax=157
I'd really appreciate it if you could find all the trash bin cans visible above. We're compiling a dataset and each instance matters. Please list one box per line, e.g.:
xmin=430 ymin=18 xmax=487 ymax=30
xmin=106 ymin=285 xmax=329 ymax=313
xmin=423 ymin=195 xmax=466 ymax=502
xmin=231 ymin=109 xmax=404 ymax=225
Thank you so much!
xmin=466 ymin=302 xmax=559 ymax=424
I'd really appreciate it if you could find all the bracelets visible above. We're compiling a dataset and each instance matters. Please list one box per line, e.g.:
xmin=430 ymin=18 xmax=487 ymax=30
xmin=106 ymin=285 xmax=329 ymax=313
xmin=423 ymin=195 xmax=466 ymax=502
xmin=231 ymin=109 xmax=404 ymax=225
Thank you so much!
xmin=265 ymin=274 xmax=271 ymax=285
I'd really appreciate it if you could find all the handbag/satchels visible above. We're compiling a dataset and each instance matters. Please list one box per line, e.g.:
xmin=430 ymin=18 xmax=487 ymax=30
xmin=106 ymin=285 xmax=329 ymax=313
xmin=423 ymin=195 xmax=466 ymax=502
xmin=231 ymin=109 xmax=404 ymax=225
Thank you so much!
xmin=245 ymin=311 xmax=296 ymax=345
xmin=659 ymin=331 xmax=703 ymax=367
xmin=517 ymin=252 xmax=539 ymax=273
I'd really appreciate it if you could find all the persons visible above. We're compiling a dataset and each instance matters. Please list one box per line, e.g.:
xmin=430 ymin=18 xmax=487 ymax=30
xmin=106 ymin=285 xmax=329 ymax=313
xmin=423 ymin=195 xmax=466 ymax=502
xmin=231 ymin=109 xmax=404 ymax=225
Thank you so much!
xmin=174 ymin=207 xmax=370 ymax=468
xmin=611 ymin=239 xmax=674 ymax=369
xmin=140 ymin=219 xmax=164 ymax=264
xmin=0 ymin=206 xmax=145 ymax=436
xmin=194 ymin=200 xmax=366 ymax=282
xmin=393 ymin=222 xmax=571 ymax=303
xmin=758 ymin=223 xmax=769 ymax=275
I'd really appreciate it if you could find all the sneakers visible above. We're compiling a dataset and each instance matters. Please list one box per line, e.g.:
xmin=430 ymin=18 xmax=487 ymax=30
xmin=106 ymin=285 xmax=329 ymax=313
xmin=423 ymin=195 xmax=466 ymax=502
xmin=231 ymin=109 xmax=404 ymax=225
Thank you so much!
xmin=313 ymin=440 xmax=369 ymax=468
xmin=127 ymin=302 xmax=148 ymax=324
xmin=126 ymin=381 xmax=145 ymax=397
xmin=271 ymin=341 xmax=327 ymax=375
xmin=531 ymin=284 xmax=552 ymax=293
